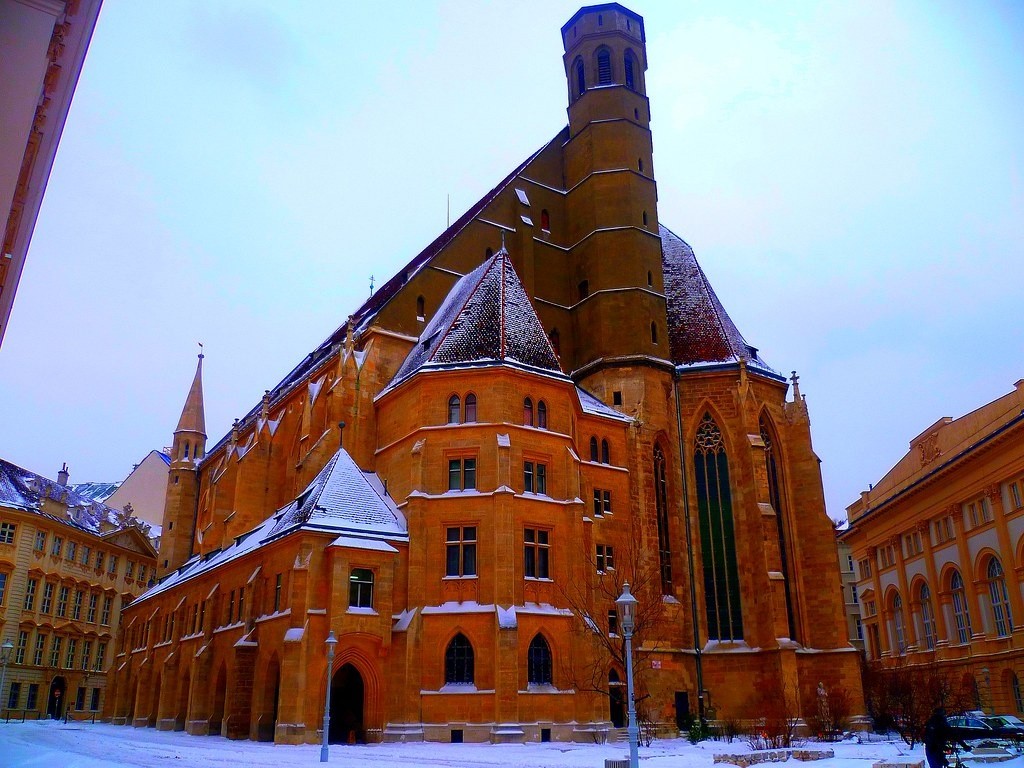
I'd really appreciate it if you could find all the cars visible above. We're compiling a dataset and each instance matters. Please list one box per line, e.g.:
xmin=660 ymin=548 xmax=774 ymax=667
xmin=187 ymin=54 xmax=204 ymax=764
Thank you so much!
xmin=946 ymin=711 xmax=1024 ymax=740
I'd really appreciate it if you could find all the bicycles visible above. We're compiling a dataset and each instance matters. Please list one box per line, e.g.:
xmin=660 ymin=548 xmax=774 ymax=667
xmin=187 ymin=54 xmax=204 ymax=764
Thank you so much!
xmin=943 ymin=746 xmax=969 ymax=768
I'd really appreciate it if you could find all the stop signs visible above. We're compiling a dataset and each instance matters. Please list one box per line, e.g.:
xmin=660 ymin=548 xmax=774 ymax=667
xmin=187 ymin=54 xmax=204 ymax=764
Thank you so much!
xmin=55 ymin=690 xmax=61 ymax=698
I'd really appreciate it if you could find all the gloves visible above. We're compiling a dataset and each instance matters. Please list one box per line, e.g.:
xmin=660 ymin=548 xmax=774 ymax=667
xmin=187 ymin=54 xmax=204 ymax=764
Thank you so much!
xmin=963 ymin=746 xmax=972 ymax=752
xmin=943 ymin=746 xmax=949 ymax=751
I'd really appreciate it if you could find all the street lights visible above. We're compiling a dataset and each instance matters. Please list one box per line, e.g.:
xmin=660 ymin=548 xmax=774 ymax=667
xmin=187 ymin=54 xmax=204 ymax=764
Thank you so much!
xmin=616 ymin=580 xmax=642 ymax=768
xmin=671 ymin=367 xmax=706 ymax=737
xmin=0 ymin=638 xmax=14 ymax=707
xmin=321 ymin=632 xmax=339 ymax=761
